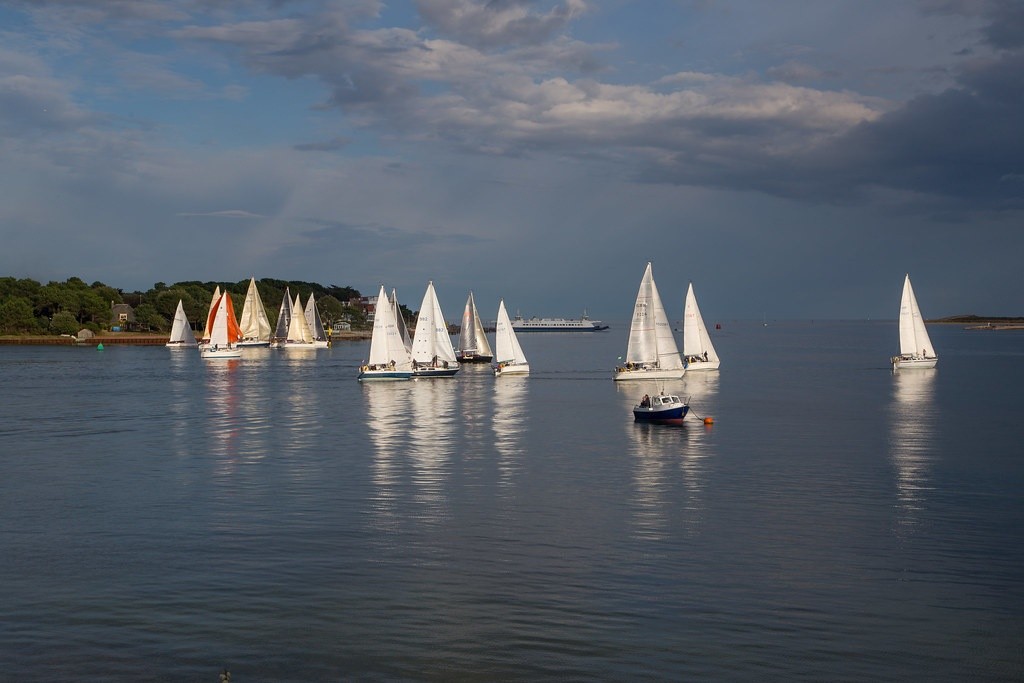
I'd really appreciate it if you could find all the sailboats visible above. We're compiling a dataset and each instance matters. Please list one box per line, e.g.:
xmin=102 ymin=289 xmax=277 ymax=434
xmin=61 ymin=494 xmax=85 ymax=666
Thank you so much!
xmin=357 ymin=284 xmax=415 ymax=381
xmin=266 ymin=284 xmax=329 ymax=349
xmin=611 ymin=261 xmax=688 ymax=382
xmin=165 ymin=298 xmax=198 ymax=348
xmin=407 ymin=279 xmax=462 ymax=379
xmin=891 ymin=272 xmax=942 ymax=369
xmin=677 ymin=281 xmax=724 ymax=371
xmin=452 ymin=289 xmax=496 ymax=363
xmin=491 ymin=297 xmax=530 ymax=377
xmin=234 ymin=276 xmax=274 ymax=347
xmin=196 ymin=284 xmax=245 ymax=359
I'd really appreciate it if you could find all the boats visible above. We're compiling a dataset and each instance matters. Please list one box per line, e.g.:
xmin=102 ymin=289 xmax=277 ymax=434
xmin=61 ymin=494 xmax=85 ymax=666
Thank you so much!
xmin=510 ymin=308 xmax=609 ymax=333
xmin=631 ymin=373 xmax=693 ymax=426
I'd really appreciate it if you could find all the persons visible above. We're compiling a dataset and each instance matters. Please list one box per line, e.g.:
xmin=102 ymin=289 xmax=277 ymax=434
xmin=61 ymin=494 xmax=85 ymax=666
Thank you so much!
xmin=215 ymin=344 xmax=217 ymax=349
xmin=923 ymin=349 xmax=926 ymax=355
xmin=626 ymin=362 xmax=633 ymax=371
xmin=684 ymin=357 xmax=688 ymax=362
xmin=692 ymin=357 xmax=695 ymax=362
xmin=364 ymin=364 xmax=376 ymax=371
xmin=633 ymin=363 xmax=639 ymax=369
xmin=391 ymin=360 xmax=396 ymax=366
xmin=704 ymin=351 xmax=708 ymax=361
xmin=498 ymin=362 xmax=510 ymax=368
xmin=413 ymin=359 xmax=417 ymax=366
xmin=641 ymin=395 xmax=649 ymax=406
xmin=444 ymin=361 xmax=448 ymax=368
